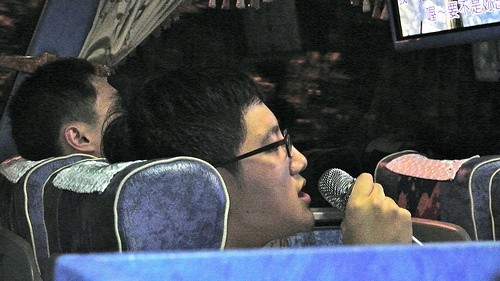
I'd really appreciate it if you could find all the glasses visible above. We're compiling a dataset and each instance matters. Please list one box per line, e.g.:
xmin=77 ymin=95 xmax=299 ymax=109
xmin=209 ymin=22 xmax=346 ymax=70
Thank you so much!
xmin=215 ymin=129 xmax=293 ymax=168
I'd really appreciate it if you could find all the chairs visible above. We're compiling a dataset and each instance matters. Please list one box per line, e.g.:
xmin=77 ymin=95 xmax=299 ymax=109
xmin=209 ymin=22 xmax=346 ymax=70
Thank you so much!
xmin=0 ymin=154 xmax=229 ymax=281
xmin=373 ymin=149 xmax=500 ymax=240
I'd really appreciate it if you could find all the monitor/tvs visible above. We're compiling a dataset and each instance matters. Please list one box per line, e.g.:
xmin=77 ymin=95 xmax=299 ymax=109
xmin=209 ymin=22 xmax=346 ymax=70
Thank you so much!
xmin=387 ymin=0 xmax=500 ymax=52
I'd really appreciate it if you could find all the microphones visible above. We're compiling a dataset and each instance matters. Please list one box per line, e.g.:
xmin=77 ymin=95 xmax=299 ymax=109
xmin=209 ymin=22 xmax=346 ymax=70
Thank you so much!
xmin=318 ymin=168 xmax=422 ymax=245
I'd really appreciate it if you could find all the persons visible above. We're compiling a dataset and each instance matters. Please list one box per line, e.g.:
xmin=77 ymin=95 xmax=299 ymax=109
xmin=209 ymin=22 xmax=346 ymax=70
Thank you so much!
xmin=101 ymin=66 xmax=413 ymax=249
xmin=8 ymin=58 xmax=126 ymax=160
